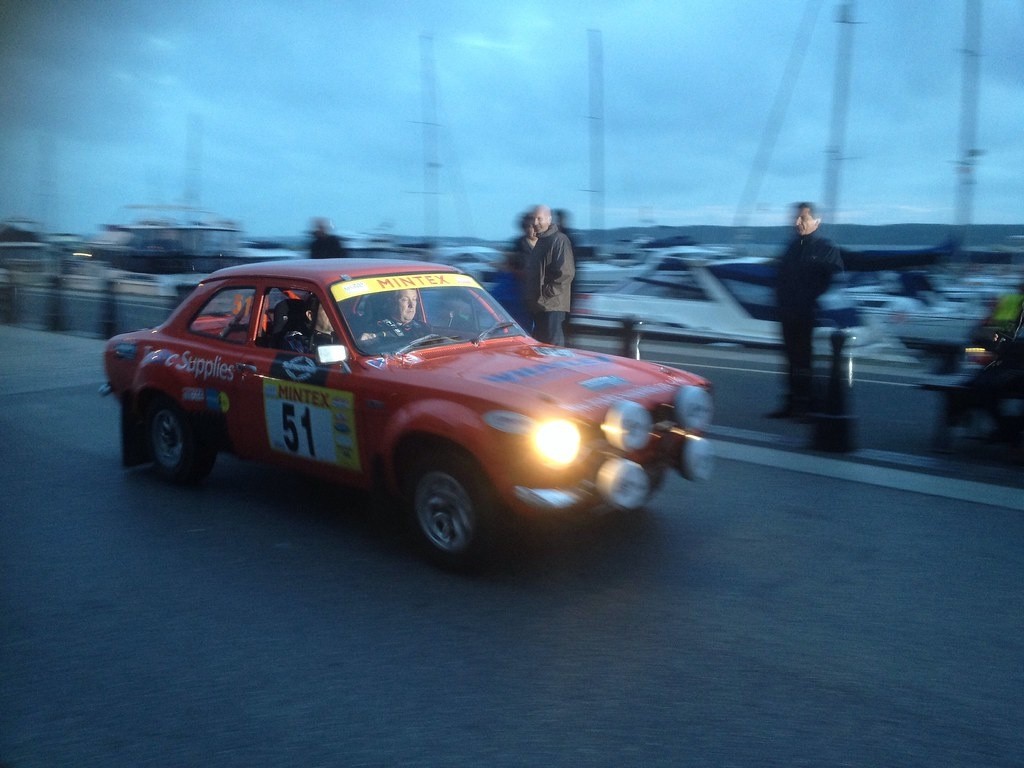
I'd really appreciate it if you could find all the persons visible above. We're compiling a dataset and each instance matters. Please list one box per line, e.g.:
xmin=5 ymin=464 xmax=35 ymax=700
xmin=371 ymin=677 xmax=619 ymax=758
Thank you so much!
xmin=762 ymin=201 xmax=837 ymax=420
xmin=507 ymin=204 xmax=577 ymax=347
xmin=376 ymin=290 xmax=437 ymax=337
xmin=310 ymin=219 xmax=346 ymax=259
xmin=281 ymin=292 xmax=378 ymax=353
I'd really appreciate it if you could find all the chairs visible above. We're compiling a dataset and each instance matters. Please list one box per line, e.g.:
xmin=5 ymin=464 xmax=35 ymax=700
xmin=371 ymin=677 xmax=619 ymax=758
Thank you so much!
xmin=263 ymin=298 xmax=306 ymax=348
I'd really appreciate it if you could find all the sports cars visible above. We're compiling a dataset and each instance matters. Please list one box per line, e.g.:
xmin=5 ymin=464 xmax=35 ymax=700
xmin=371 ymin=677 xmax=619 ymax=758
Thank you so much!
xmin=101 ymin=254 xmax=713 ymax=572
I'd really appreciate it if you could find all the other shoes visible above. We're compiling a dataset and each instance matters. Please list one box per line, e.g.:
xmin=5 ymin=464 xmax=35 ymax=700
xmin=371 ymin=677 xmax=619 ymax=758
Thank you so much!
xmin=766 ymin=394 xmax=806 ymax=418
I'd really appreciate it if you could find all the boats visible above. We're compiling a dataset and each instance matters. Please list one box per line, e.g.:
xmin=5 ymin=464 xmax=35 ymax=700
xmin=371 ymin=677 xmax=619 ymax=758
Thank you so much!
xmin=374 ymin=0 xmax=1024 ymax=378
